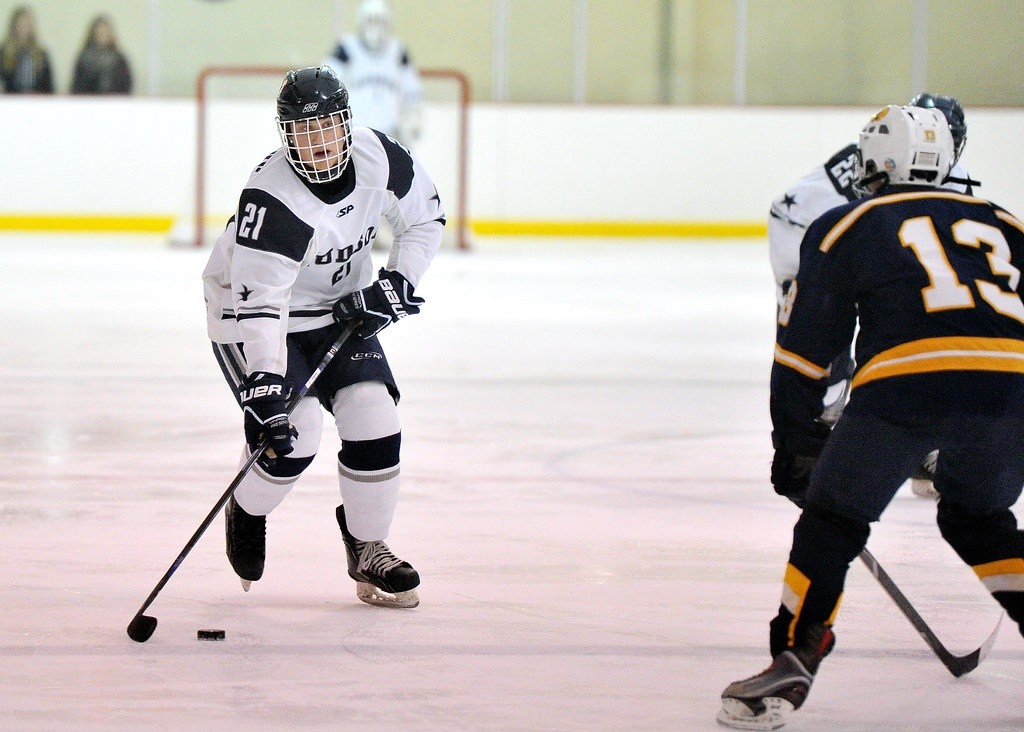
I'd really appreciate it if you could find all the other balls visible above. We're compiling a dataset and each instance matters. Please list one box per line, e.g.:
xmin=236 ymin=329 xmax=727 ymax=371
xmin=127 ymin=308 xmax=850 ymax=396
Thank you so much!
xmin=197 ymin=629 xmax=225 ymax=641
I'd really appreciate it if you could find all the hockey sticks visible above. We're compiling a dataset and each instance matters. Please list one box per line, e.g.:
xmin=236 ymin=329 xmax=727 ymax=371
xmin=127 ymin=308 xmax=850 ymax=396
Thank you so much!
xmin=858 ymin=545 xmax=1005 ymax=679
xmin=127 ymin=322 xmax=355 ymax=644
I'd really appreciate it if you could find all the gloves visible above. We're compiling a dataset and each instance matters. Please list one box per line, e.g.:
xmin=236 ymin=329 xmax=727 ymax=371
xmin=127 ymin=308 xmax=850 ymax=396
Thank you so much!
xmin=770 ymin=427 xmax=831 ymax=504
xmin=331 ymin=267 xmax=423 ymax=341
xmin=238 ymin=372 xmax=298 ymax=456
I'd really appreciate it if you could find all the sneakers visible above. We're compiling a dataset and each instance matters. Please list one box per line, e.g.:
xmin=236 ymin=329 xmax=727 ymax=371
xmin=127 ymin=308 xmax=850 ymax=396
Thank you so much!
xmin=716 ymin=650 xmax=835 ymax=730
xmin=336 ymin=503 xmax=420 ymax=608
xmin=910 ymin=449 xmax=940 ymax=498
xmin=224 ymin=494 xmax=266 ymax=593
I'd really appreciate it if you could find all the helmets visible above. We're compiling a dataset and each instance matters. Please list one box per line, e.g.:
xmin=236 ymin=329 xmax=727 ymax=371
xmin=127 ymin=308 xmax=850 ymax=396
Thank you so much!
xmin=277 ymin=65 xmax=354 ymax=181
xmin=856 ymin=102 xmax=955 ymax=192
xmin=908 ymin=91 xmax=967 ymax=160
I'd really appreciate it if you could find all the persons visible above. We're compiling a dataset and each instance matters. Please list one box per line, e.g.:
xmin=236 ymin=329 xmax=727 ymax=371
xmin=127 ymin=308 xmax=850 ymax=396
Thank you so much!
xmin=0 ymin=5 xmax=56 ymax=95
xmin=324 ymin=0 xmax=425 ymax=148
xmin=203 ymin=63 xmax=448 ymax=607
xmin=715 ymin=92 xmax=1024 ymax=732
xmin=69 ymin=14 xmax=133 ymax=97
